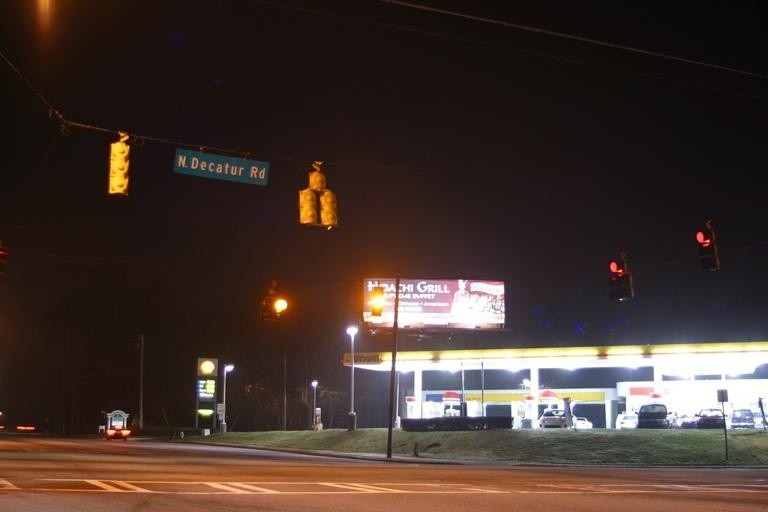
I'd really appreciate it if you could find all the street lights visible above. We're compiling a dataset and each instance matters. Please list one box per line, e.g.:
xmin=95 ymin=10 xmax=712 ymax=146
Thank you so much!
xmin=310 ymin=379 xmax=319 ymax=431
xmin=220 ymin=363 xmax=234 ymax=432
xmin=345 ymin=325 xmax=358 ymax=430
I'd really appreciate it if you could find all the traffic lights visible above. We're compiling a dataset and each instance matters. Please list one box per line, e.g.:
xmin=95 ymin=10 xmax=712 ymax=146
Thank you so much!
xmin=694 ymin=219 xmax=719 ymax=272
xmin=370 ymin=284 xmax=385 ymax=318
xmin=257 ymin=293 xmax=287 ymax=322
xmin=606 ymin=248 xmax=634 ymax=303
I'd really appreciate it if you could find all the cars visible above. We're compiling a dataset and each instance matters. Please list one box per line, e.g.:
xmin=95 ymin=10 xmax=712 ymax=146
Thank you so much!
xmin=615 ymin=402 xmax=757 ymax=430
xmin=538 ymin=409 xmax=573 ymax=429
xmin=573 ymin=416 xmax=596 ymax=429
xmin=105 ymin=424 xmax=128 ymax=441
